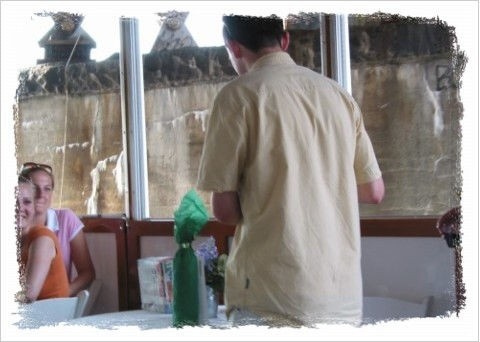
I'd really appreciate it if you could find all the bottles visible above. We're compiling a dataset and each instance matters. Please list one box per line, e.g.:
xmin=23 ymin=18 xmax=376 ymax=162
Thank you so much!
xmin=173 ymin=242 xmax=199 ymax=326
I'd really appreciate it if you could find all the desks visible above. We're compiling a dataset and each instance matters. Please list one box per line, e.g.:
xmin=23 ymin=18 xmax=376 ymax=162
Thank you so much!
xmin=62 ymin=292 xmax=431 ymax=329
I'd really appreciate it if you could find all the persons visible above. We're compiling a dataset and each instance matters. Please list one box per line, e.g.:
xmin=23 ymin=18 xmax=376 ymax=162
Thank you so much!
xmin=19 ymin=161 xmax=96 ymax=299
xmin=15 ymin=174 xmax=71 ymax=305
xmin=197 ymin=14 xmax=385 ymax=329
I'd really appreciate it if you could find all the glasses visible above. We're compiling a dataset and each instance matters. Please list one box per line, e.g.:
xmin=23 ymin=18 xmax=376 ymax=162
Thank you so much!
xmin=21 ymin=162 xmax=53 ymax=171
